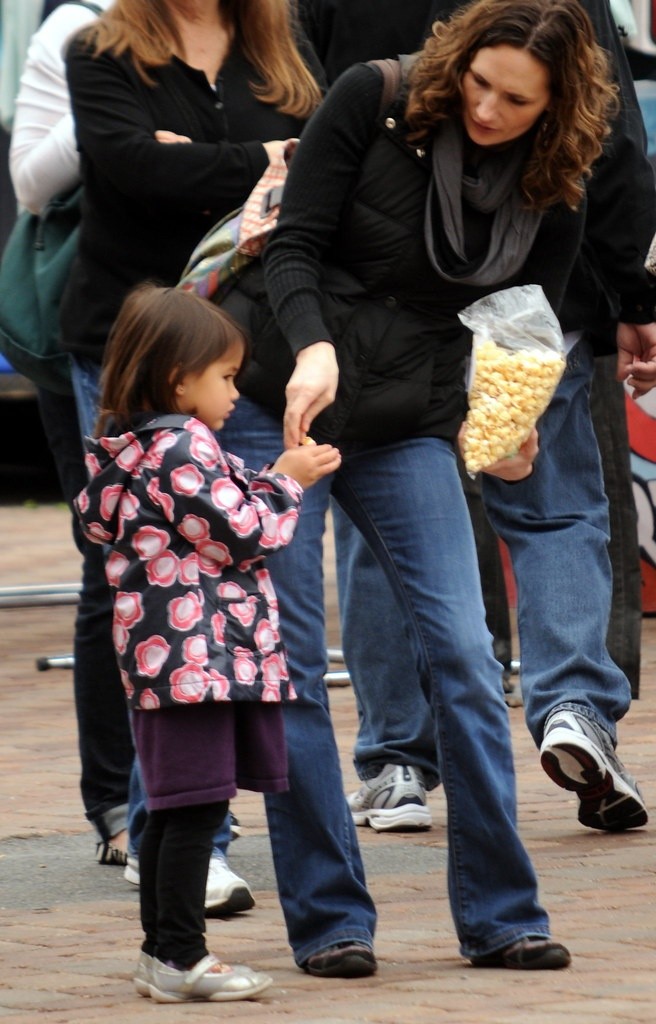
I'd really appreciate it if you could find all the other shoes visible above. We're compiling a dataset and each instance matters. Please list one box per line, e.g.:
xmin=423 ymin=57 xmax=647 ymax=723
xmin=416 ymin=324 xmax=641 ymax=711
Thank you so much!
xmin=305 ymin=943 xmax=378 ymax=978
xmin=149 ymin=956 xmax=273 ymax=1002
xmin=95 ymin=842 xmax=128 ymax=865
xmin=133 ymin=950 xmax=154 ymax=997
xmin=471 ymin=937 xmax=572 ymax=970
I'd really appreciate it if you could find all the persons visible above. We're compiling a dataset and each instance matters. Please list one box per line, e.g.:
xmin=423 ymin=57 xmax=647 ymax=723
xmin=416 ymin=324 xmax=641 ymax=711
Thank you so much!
xmin=68 ymin=284 xmax=345 ymax=1007
xmin=57 ymin=0 xmax=331 ymax=915
xmin=330 ymin=0 xmax=656 ymax=836
xmin=205 ymin=0 xmax=625 ymax=981
xmin=6 ymin=0 xmax=145 ymax=870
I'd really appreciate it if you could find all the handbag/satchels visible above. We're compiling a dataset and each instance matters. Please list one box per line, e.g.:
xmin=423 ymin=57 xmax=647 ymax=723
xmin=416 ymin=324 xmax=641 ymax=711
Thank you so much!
xmin=174 ymin=159 xmax=289 ymax=300
xmin=0 ymin=176 xmax=84 ymax=400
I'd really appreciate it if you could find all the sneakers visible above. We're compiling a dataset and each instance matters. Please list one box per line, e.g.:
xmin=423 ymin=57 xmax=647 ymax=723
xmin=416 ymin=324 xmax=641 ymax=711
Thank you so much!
xmin=122 ymin=852 xmax=256 ymax=916
xmin=343 ymin=763 xmax=432 ymax=832
xmin=539 ymin=710 xmax=648 ymax=830
